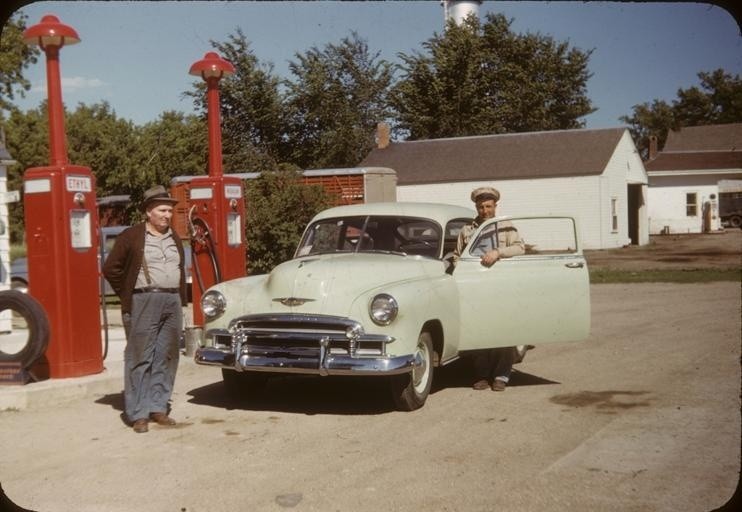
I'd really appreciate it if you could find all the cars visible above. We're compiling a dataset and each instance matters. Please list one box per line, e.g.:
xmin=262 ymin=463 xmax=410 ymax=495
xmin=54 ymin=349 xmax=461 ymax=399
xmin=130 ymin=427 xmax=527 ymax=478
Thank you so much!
xmin=194 ymin=199 xmax=590 ymax=412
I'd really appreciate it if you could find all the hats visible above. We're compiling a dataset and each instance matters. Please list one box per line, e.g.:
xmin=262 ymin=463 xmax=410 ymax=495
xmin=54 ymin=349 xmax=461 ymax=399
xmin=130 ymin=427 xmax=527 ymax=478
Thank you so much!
xmin=137 ymin=186 xmax=179 ymax=212
xmin=471 ymin=187 xmax=499 ymax=202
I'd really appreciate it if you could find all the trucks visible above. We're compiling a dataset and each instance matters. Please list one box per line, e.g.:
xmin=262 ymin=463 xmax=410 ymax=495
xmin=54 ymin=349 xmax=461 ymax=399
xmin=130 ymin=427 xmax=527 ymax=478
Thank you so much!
xmin=719 ymin=190 xmax=742 ymax=228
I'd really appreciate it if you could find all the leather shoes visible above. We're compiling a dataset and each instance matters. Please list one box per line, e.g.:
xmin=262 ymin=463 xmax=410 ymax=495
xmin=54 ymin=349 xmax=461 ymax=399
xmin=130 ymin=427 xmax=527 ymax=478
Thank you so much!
xmin=150 ymin=413 xmax=175 ymax=425
xmin=492 ymin=380 xmax=505 ymax=390
xmin=474 ymin=380 xmax=488 ymax=389
xmin=134 ymin=419 xmax=148 ymax=432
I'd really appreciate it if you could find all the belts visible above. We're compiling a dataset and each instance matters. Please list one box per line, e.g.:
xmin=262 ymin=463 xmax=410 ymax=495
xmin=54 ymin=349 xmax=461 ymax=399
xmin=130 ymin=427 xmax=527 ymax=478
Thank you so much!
xmin=134 ymin=286 xmax=181 ymax=293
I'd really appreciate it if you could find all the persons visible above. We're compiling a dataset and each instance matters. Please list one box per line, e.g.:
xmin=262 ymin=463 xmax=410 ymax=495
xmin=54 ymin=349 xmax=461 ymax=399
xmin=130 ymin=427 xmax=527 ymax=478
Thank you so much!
xmin=101 ymin=185 xmax=188 ymax=435
xmin=452 ymin=186 xmax=526 ymax=392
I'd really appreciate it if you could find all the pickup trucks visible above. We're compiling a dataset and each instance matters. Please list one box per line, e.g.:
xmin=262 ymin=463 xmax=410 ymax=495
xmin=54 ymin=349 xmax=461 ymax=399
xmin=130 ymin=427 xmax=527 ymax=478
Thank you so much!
xmin=10 ymin=225 xmax=191 ymax=298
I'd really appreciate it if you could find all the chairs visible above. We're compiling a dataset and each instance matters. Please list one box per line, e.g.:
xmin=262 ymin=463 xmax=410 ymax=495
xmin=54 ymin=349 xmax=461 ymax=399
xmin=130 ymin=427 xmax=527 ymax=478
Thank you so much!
xmin=338 ymin=235 xmax=439 ymax=258
